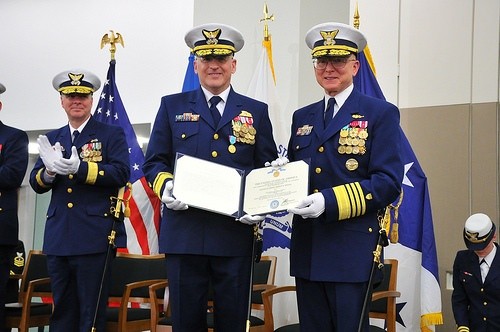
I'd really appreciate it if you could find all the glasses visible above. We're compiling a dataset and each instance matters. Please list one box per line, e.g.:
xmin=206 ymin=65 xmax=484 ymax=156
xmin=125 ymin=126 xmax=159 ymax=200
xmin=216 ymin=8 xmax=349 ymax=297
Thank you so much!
xmin=195 ymin=55 xmax=232 ymax=65
xmin=312 ymin=58 xmax=356 ymax=69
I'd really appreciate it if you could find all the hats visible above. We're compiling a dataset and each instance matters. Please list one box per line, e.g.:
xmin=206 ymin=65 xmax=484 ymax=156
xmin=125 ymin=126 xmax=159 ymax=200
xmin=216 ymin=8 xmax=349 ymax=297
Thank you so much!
xmin=0 ymin=83 xmax=6 ymax=94
xmin=306 ymin=22 xmax=367 ymax=56
xmin=463 ymin=213 xmax=496 ymax=250
xmin=184 ymin=23 xmax=244 ymax=57
xmin=52 ymin=70 xmax=101 ymax=95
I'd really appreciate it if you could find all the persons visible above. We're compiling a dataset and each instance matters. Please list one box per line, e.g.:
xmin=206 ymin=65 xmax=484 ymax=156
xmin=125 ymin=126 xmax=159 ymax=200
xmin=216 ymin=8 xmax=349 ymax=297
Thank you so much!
xmin=0 ymin=84 xmax=29 ymax=332
xmin=451 ymin=214 xmax=500 ymax=332
xmin=265 ymin=22 xmax=403 ymax=332
xmin=30 ymin=68 xmax=129 ymax=332
xmin=8 ymin=240 xmax=25 ymax=303
xmin=142 ymin=23 xmax=279 ymax=332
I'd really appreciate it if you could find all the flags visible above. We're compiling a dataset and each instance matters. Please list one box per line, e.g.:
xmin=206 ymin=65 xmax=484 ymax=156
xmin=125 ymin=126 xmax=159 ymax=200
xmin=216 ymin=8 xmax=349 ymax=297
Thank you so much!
xmin=245 ymin=38 xmax=299 ymax=332
xmin=261 ymin=44 xmax=442 ymax=332
xmin=182 ymin=54 xmax=200 ymax=93
xmin=92 ymin=60 xmax=160 ymax=310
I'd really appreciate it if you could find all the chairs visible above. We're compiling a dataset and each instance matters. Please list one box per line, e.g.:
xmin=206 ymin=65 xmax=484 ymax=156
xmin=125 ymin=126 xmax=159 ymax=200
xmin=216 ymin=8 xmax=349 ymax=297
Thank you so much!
xmin=4 ymin=249 xmax=401 ymax=332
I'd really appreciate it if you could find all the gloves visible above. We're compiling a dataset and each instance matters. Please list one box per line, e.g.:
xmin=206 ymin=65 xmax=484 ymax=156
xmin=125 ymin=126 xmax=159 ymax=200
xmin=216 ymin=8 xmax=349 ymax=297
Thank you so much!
xmin=287 ymin=192 xmax=325 ymax=219
xmin=52 ymin=146 xmax=81 ymax=175
xmin=161 ymin=180 xmax=189 ymax=210
xmin=264 ymin=157 xmax=289 ymax=167
xmin=37 ymin=134 xmax=63 ymax=171
xmin=239 ymin=215 xmax=266 ymax=225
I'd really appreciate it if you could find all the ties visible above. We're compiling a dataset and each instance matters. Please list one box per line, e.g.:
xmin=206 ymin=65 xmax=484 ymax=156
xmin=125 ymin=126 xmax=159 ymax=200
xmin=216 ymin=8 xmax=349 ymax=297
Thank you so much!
xmin=480 ymin=262 xmax=489 ymax=284
xmin=72 ymin=131 xmax=79 ymax=144
xmin=209 ymin=96 xmax=222 ymax=129
xmin=324 ymin=98 xmax=335 ymax=129
